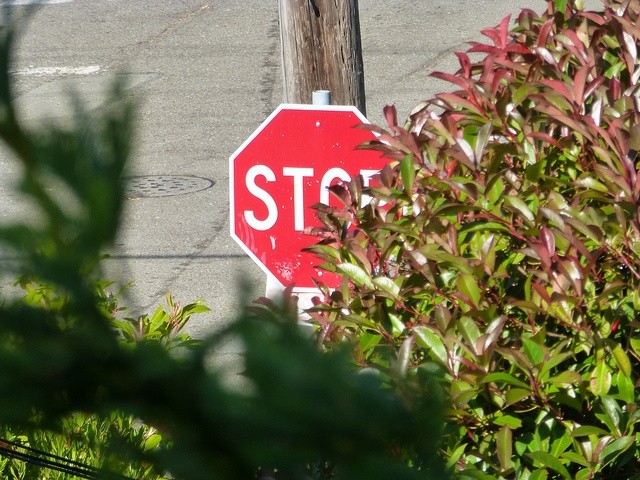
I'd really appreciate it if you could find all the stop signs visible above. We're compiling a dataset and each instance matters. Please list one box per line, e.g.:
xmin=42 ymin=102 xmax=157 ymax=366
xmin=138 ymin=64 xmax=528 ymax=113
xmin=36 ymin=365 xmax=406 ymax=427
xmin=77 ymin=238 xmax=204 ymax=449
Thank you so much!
xmin=228 ymin=103 xmax=405 ymax=292
